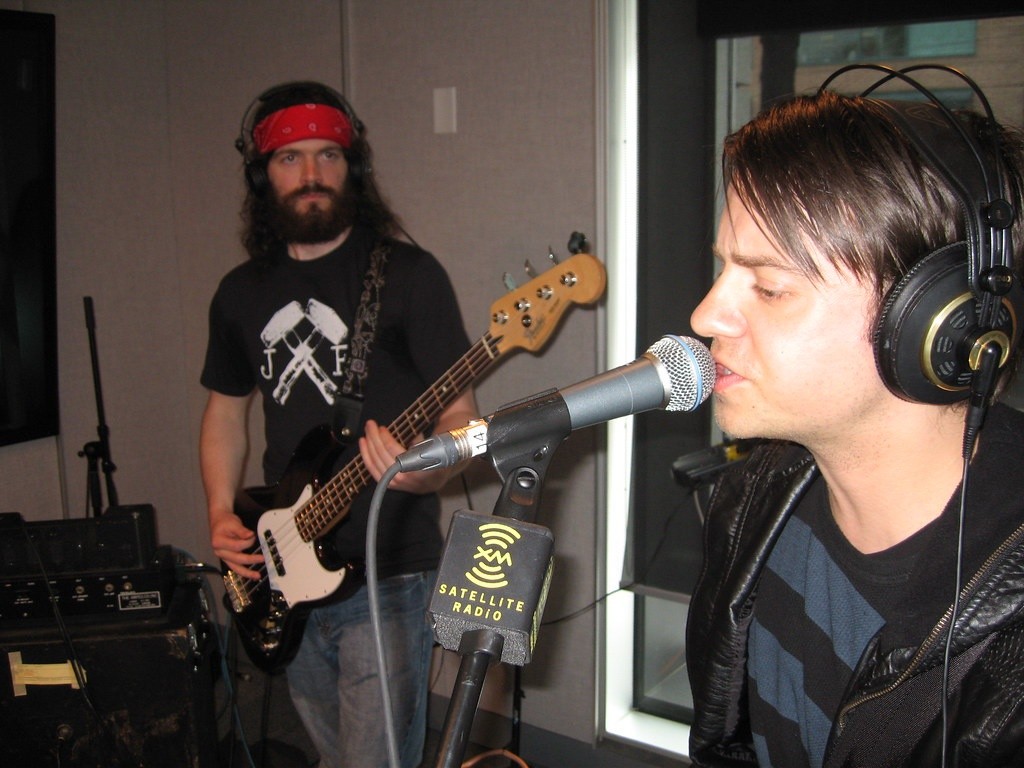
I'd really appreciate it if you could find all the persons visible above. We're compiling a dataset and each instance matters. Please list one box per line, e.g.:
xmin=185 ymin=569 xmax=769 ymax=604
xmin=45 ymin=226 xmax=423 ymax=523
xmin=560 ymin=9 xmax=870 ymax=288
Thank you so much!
xmin=691 ymin=90 xmax=1024 ymax=768
xmin=198 ymin=80 xmax=479 ymax=767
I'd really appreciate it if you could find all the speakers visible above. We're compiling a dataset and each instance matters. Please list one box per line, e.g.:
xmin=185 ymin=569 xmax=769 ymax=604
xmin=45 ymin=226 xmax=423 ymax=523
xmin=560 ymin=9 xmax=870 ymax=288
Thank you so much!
xmin=0 ymin=614 xmax=219 ymax=768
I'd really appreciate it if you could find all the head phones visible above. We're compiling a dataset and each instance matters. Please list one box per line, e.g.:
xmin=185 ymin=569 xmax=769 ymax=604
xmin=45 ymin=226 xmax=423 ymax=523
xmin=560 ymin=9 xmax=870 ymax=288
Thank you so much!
xmin=813 ymin=64 xmax=1024 ymax=405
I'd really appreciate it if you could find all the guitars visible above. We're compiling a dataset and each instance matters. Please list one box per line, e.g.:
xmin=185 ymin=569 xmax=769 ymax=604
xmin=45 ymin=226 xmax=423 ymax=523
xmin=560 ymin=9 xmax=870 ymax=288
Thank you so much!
xmin=221 ymin=230 xmax=608 ymax=675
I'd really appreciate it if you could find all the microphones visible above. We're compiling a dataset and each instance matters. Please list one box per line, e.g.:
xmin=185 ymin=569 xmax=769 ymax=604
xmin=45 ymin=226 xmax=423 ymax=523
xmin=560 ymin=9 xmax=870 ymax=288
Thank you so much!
xmin=395 ymin=335 xmax=718 ymax=472
